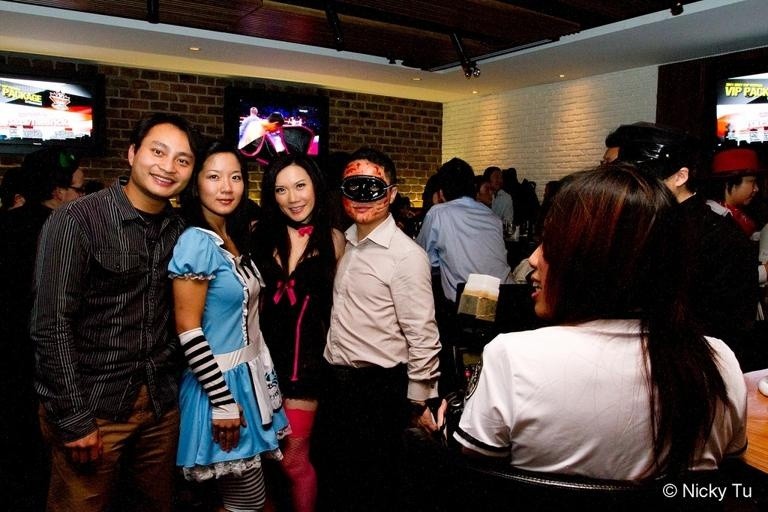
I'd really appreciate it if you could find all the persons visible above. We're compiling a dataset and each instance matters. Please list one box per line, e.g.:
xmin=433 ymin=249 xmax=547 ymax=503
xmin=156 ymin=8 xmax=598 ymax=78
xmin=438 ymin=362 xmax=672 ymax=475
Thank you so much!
xmin=318 ymin=139 xmax=440 ymax=512
xmin=166 ymin=137 xmax=294 ymax=511
xmin=0 ymin=105 xmax=767 ymax=408
xmin=448 ymin=158 xmax=748 ymax=510
xmin=242 ymin=149 xmax=348 ymax=511
xmin=25 ymin=111 xmax=209 ymax=512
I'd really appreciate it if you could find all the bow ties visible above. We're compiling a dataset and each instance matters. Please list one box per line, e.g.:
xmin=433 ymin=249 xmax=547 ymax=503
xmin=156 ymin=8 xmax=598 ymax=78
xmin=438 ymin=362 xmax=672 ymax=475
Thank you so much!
xmin=287 ymin=219 xmax=315 ymax=236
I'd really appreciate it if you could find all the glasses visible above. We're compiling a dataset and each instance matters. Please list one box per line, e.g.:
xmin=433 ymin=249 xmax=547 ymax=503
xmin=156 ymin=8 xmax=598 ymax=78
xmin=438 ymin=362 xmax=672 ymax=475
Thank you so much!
xmin=55 ymin=185 xmax=86 ymax=193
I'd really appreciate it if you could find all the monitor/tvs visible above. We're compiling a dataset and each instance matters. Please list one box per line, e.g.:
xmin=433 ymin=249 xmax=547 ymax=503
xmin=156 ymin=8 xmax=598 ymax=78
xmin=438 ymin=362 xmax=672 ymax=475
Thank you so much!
xmin=0 ymin=67 xmax=108 ymax=154
xmin=707 ymin=61 xmax=768 ymax=149
xmin=224 ymin=86 xmax=330 ymax=165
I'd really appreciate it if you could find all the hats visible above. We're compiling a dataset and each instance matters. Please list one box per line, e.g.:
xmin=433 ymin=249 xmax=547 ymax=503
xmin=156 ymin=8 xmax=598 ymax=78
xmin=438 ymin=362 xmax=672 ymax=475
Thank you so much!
xmin=18 ymin=141 xmax=82 ymax=196
xmin=713 ymin=150 xmax=759 ymax=172
